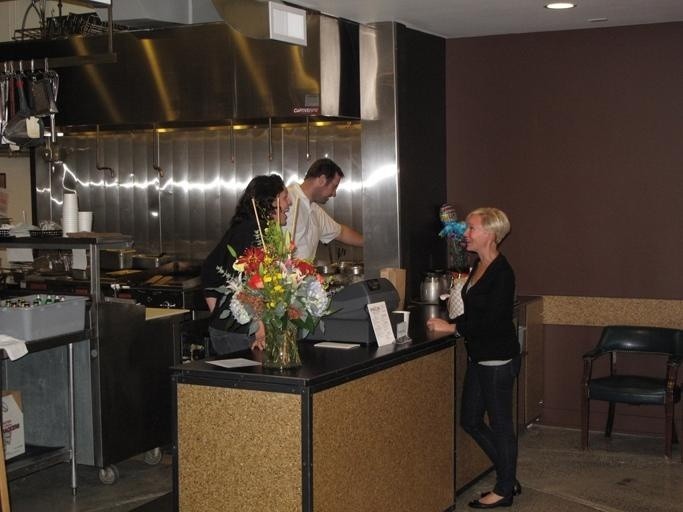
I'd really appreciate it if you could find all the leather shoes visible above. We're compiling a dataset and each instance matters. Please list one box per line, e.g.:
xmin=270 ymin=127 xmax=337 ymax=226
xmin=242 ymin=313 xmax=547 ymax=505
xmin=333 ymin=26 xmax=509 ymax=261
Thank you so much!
xmin=469 ymin=480 xmax=521 ymax=508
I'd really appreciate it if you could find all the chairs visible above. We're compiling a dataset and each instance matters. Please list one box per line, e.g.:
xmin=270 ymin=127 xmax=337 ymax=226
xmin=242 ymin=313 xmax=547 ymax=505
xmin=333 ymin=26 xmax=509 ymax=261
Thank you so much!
xmin=577 ymin=324 xmax=682 ymax=460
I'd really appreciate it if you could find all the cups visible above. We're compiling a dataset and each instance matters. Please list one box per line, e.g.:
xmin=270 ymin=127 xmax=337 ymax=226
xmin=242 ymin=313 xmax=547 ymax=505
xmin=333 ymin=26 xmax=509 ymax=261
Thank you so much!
xmin=62 ymin=193 xmax=93 ymax=238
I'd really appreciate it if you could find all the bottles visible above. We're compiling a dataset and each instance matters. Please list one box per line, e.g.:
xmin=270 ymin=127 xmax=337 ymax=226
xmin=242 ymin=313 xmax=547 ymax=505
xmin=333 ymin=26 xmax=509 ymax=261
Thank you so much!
xmin=5 ymin=293 xmax=66 ymax=308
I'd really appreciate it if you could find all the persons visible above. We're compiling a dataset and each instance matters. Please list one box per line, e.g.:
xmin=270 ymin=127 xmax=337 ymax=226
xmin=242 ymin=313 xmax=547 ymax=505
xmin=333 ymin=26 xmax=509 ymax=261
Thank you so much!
xmin=275 ymin=158 xmax=363 ymax=266
xmin=425 ymin=206 xmax=521 ymax=510
xmin=199 ymin=173 xmax=292 ymax=357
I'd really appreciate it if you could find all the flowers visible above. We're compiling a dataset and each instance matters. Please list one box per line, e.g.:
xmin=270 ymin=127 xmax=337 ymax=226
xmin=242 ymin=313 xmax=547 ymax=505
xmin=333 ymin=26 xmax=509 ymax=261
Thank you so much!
xmin=214 ymin=191 xmax=344 ymax=365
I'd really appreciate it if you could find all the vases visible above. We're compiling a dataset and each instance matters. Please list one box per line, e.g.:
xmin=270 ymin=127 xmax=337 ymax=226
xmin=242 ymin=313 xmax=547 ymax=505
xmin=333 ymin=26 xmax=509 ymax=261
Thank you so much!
xmin=264 ymin=317 xmax=302 ymax=370
